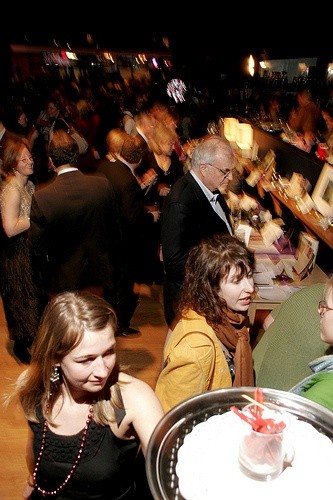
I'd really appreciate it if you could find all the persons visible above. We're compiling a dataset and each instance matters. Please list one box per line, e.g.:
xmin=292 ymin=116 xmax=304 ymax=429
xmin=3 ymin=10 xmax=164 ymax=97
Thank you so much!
xmin=1 ymin=291 xmax=164 ymax=500
xmin=155 ymin=235 xmax=254 ymax=416
xmin=0 ymin=59 xmax=333 ymax=363
xmin=251 ymin=281 xmax=333 ymax=391
xmin=287 ymin=274 xmax=333 ymax=412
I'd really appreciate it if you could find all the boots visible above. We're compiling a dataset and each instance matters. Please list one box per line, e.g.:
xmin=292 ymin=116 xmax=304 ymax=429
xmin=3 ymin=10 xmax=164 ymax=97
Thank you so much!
xmin=13 ymin=340 xmax=32 ymax=365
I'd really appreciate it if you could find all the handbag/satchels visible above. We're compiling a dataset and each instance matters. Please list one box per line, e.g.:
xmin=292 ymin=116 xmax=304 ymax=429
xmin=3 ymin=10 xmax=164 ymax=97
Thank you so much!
xmin=57 ymin=118 xmax=88 ymax=156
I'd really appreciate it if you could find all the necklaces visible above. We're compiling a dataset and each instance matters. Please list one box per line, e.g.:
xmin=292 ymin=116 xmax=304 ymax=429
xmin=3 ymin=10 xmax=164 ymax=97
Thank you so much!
xmin=33 ymin=401 xmax=93 ymax=496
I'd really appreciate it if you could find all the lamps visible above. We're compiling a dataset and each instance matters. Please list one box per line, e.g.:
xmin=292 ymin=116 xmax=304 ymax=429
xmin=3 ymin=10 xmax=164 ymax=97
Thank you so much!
xmin=224 ymin=119 xmax=253 ymax=160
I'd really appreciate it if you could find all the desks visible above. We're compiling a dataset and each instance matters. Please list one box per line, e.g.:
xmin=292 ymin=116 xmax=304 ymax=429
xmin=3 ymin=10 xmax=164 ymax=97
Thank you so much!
xmin=237 ymin=253 xmax=329 ymax=326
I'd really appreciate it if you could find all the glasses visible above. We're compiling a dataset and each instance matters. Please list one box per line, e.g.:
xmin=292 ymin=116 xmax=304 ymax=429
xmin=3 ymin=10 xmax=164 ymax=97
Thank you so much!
xmin=19 ymin=157 xmax=35 ymax=163
xmin=201 ymin=162 xmax=233 ymax=178
xmin=318 ymin=300 xmax=333 ymax=315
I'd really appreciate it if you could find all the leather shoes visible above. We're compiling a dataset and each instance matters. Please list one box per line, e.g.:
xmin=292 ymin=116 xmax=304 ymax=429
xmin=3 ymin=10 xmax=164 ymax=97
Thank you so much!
xmin=115 ymin=326 xmax=141 ymax=337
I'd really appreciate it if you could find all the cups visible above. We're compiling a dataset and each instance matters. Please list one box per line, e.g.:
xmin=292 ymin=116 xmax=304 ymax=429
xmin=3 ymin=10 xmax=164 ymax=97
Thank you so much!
xmin=239 ymin=402 xmax=289 ymax=476
